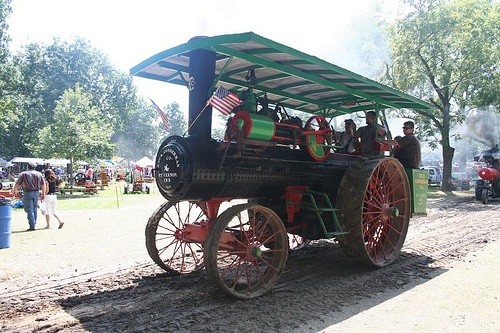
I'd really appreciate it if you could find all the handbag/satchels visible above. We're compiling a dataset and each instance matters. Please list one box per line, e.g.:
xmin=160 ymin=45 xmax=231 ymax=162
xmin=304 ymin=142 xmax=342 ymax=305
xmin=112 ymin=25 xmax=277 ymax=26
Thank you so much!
xmin=40 ymin=199 xmax=47 ymax=215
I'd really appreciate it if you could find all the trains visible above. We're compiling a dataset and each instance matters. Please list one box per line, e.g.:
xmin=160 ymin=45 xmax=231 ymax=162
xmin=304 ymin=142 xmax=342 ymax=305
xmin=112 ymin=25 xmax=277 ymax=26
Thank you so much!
xmin=473 ymin=144 xmax=500 ymax=205
xmin=130 ymin=32 xmax=436 ymax=301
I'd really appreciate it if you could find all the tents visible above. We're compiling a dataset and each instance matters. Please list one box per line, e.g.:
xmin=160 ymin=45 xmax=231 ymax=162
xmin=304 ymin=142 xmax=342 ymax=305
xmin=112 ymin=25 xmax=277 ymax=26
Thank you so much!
xmin=135 ymin=156 xmax=155 ymax=175
xmin=0 ymin=158 xmax=71 ymax=171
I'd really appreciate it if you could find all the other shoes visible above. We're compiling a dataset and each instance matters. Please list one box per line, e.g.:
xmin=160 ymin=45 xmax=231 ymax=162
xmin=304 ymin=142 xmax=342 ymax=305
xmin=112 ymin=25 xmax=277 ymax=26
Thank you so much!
xmin=27 ymin=228 xmax=35 ymax=231
xmin=58 ymin=222 xmax=64 ymax=229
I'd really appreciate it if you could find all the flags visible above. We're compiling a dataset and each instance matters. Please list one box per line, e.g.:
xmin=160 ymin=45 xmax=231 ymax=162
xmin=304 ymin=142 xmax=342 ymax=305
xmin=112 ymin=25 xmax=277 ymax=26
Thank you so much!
xmin=207 ymin=86 xmax=241 ymax=116
xmin=150 ymin=100 xmax=170 ymax=132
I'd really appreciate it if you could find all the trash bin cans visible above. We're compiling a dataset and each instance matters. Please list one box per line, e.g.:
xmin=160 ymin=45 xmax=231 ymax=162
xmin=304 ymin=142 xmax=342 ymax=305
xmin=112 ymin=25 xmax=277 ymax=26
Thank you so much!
xmin=0 ymin=199 xmax=12 ymax=249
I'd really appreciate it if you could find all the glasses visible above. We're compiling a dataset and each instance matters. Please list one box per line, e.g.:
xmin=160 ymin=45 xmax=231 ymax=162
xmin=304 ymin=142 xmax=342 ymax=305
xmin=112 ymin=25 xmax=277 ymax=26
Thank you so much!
xmin=402 ymin=127 xmax=412 ymax=129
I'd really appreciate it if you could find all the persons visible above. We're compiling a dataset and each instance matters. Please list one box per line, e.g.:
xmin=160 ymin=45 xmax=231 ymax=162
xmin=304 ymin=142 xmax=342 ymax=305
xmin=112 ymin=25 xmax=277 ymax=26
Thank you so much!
xmin=12 ymin=162 xmax=46 ymax=231
xmin=40 ymin=169 xmax=64 ymax=230
xmin=331 ymin=118 xmax=359 ymax=153
xmin=354 ymin=111 xmax=386 ymax=155
xmin=375 ymin=121 xmax=422 ymax=169
xmin=0 ymin=165 xmax=155 ymax=190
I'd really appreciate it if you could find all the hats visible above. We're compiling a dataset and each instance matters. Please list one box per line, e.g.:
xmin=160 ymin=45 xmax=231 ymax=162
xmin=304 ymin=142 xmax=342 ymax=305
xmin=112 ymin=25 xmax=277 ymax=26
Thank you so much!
xmin=28 ymin=161 xmax=37 ymax=168
